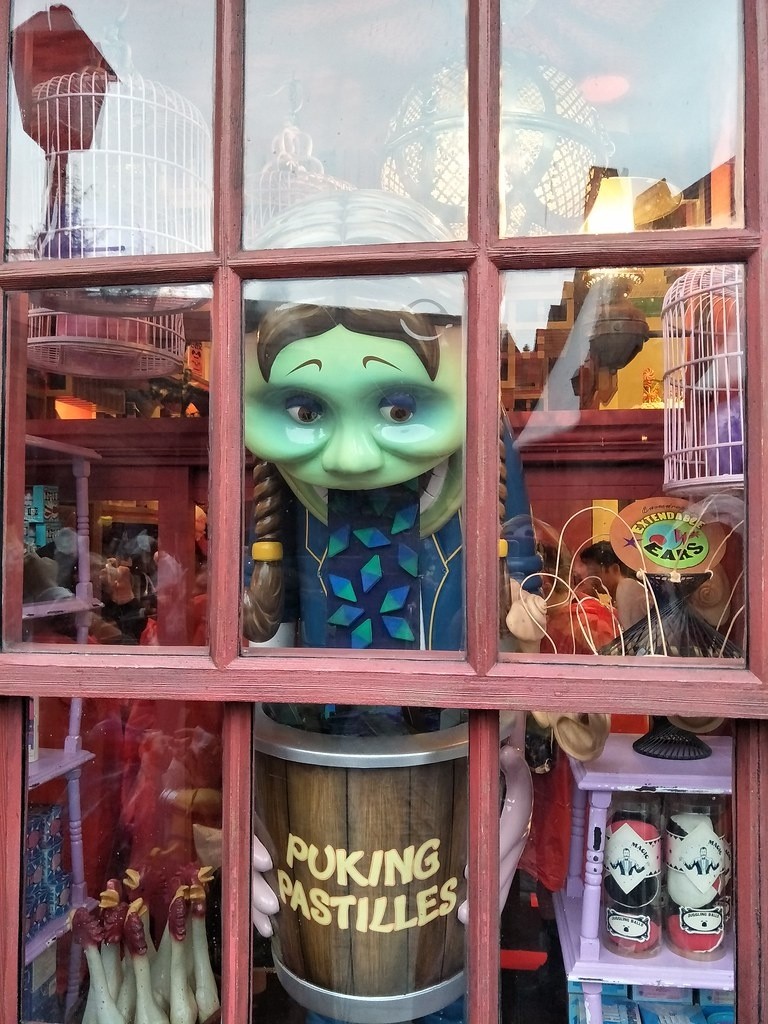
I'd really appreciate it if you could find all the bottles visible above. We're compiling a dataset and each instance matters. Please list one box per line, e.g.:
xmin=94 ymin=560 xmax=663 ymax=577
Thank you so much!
xmin=661 ymin=794 xmax=734 ymax=962
xmin=599 ymin=792 xmax=663 ymax=960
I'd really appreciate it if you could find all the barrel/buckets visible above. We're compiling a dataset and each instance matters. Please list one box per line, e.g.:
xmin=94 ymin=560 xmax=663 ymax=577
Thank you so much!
xmin=252 ymin=703 xmax=517 ymax=1024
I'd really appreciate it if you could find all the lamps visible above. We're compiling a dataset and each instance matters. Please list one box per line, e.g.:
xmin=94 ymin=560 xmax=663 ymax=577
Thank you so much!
xmin=595 ymin=570 xmax=745 ymax=763
xmin=573 ymin=142 xmax=708 ymax=368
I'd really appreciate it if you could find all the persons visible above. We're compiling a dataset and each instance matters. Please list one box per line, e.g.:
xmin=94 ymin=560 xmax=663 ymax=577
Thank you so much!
xmin=21 ymin=529 xmax=190 ymax=647
xmin=241 ymin=189 xmax=547 ymax=938
xmin=580 ymin=540 xmax=653 ymax=630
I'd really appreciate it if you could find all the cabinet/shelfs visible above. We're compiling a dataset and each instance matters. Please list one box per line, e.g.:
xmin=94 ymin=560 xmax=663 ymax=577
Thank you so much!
xmin=18 ymin=431 xmax=99 ymax=1024
xmin=550 ymin=730 xmax=736 ymax=1024
